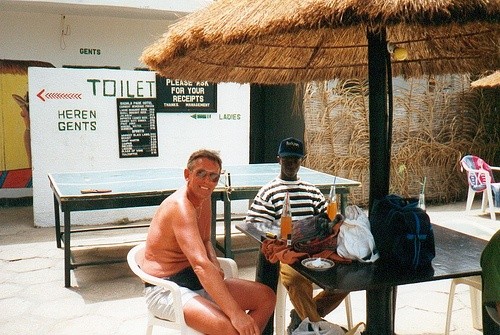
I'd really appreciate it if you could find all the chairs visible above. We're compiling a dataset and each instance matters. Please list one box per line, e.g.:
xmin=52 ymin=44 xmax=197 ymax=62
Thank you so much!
xmin=127 ymin=242 xmax=239 ymax=335
xmin=462 ymin=155 xmax=500 ymax=221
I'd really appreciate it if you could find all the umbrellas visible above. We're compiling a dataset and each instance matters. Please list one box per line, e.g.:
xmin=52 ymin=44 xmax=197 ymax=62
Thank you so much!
xmin=136 ymin=0 xmax=500 ymax=335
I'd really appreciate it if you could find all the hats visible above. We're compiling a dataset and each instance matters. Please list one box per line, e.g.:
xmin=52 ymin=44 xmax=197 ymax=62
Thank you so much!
xmin=278 ymin=137 xmax=304 ymax=158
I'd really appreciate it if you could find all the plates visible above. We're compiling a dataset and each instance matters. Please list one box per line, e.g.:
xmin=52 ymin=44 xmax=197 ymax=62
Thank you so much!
xmin=301 ymin=257 xmax=335 ymax=271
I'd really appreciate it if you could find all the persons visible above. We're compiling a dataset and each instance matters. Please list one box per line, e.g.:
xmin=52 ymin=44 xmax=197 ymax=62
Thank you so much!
xmin=12 ymin=91 xmax=32 ymax=169
xmin=140 ymin=149 xmax=276 ymax=335
xmin=245 ymin=138 xmax=352 ymax=335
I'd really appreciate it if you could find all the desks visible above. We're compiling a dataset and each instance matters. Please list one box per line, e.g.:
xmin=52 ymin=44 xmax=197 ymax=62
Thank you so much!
xmin=234 ymin=222 xmax=490 ymax=335
xmin=48 ymin=162 xmax=361 ymax=289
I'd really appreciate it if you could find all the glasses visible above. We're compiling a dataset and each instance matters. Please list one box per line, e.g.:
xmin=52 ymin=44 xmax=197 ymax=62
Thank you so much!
xmin=188 ymin=167 xmax=220 ymax=183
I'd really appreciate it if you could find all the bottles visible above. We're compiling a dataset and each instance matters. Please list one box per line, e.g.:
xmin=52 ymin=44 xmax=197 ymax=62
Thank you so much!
xmin=280 ymin=193 xmax=293 ymax=240
xmin=327 ymin=186 xmax=337 ymax=223
xmin=416 ymin=193 xmax=426 ymax=213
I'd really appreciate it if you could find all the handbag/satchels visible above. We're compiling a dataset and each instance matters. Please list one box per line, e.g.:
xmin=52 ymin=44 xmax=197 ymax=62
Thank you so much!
xmin=291 ymin=317 xmax=345 ymax=335
xmin=336 ymin=204 xmax=380 ymax=263
xmin=369 ymin=194 xmax=436 ymax=270
